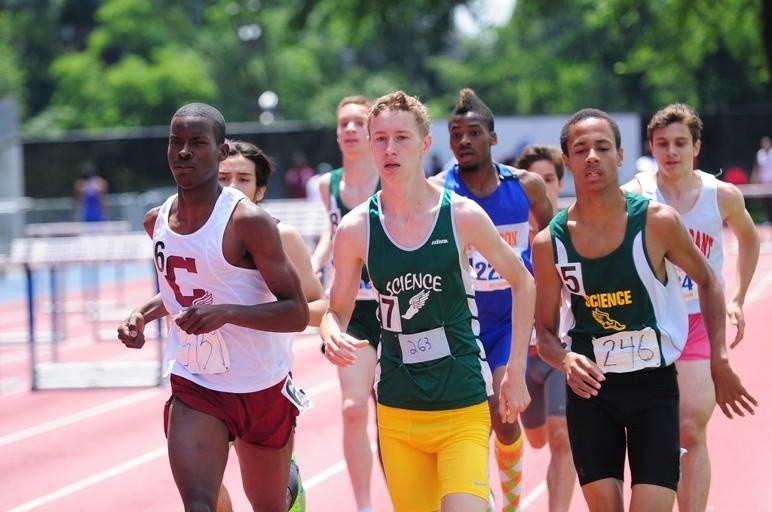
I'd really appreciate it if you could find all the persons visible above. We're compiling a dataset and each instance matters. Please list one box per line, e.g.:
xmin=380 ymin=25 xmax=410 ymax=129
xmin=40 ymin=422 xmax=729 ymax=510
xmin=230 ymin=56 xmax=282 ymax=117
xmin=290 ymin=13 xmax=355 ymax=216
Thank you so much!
xmin=425 ymin=85 xmax=553 ymax=512
xmin=752 ymin=136 xmax=772 ymax=182
xmin=312 ymin=94 xmax=388 ymax=511
xmin=620 ymin=103 xmax=762 ymax=511
xmin=288 ymin=153 xmax=333 ymax=197
xmin=219 ymin=134 xmax=328 ymax=327
xmin=118 ymin=100 xmax=308 ymax=512
xmin=511 ymin=142 xmax=580 ymax=512
xmin=75 ymin=161 xmax=110 ymax=222
xmin=530 ymin=106 xmax=758 ymax=512
xmin=318 ymin=90 xmax=537 ymax=511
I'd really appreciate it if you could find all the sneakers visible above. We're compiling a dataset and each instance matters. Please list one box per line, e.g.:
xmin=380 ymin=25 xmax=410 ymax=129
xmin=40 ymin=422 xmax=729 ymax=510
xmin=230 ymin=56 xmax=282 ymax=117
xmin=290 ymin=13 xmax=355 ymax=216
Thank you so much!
xmin=286 ymin=452 xmax=306 ymax=512
